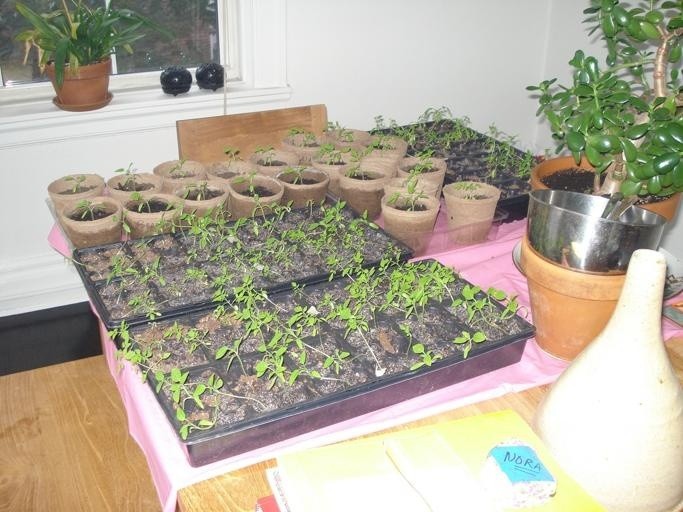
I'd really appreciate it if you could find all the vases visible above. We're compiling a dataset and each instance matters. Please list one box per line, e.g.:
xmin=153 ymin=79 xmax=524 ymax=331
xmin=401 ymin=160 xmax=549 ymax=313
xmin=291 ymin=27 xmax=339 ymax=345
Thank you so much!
xmin=521 ymin=233 xmax=627 ymax=364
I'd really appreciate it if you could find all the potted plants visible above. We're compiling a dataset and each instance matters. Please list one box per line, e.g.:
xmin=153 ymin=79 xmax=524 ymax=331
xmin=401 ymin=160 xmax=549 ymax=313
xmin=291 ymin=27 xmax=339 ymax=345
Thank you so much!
xmin=525 ymin=1 xmax=682 ymax=224
xmin=8 ymin=0 xmax=171 ymax=112
xmin=47 ymin=105 xmax=543 ymax=467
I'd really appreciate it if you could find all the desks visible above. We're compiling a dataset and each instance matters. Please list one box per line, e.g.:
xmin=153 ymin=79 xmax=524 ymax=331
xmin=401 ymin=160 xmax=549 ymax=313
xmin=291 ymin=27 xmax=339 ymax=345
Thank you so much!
xmin=97 ymin=336 xmax=683 ymax=511
